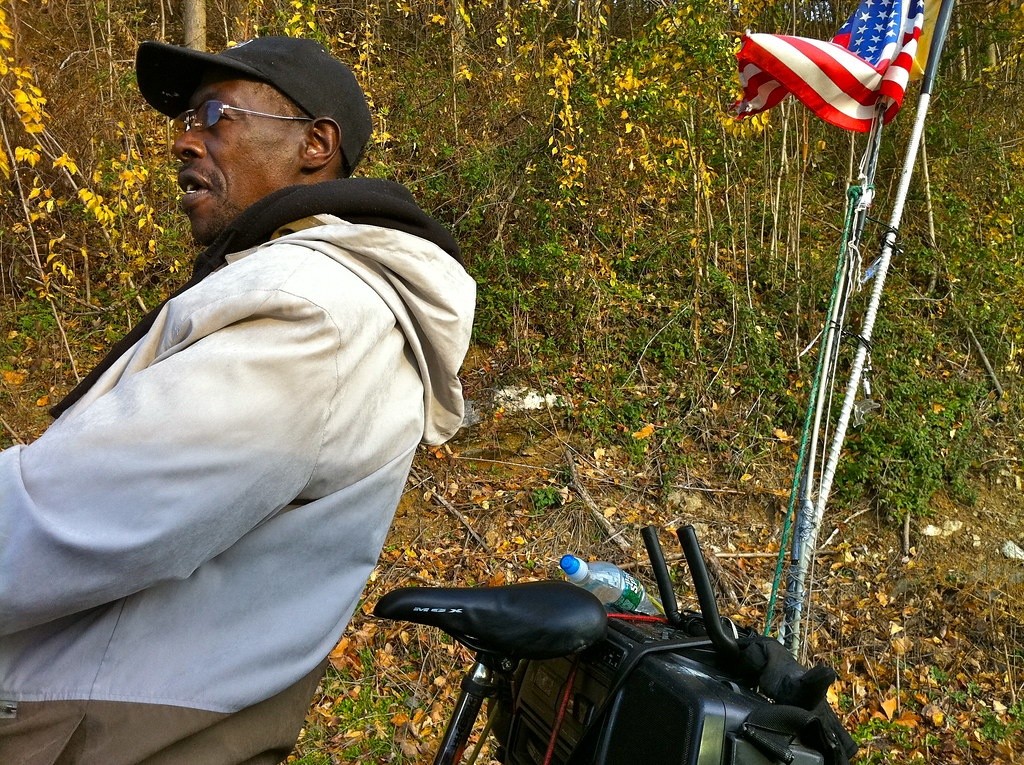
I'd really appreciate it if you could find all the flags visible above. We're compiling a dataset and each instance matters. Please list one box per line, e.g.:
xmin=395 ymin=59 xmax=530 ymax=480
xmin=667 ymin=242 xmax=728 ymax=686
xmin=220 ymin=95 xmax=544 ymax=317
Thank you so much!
xmin=730 ymin=0 xmax=943 ymax=132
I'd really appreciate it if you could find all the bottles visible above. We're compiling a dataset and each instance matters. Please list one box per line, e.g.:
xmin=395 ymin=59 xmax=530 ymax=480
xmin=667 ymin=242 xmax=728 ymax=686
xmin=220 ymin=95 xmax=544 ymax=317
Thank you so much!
xmin=561 ymin=555 xmax=663 ymax=615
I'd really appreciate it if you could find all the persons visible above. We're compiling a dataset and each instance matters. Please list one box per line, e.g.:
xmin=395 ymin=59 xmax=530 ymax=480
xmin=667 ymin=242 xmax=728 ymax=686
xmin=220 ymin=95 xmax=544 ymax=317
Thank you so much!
xmin=0 ymin=39 xmax=477 ymax=765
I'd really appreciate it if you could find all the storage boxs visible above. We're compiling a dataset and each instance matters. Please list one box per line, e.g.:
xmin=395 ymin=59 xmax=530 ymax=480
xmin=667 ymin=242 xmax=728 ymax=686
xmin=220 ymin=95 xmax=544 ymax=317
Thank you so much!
xmin=482 ymin=612 xmax=777 ymax=765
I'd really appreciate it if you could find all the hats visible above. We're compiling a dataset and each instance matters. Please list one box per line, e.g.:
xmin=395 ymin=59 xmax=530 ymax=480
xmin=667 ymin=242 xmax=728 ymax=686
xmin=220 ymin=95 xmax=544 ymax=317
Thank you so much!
xmin=135 ymin=35 xmax=371 ymax=171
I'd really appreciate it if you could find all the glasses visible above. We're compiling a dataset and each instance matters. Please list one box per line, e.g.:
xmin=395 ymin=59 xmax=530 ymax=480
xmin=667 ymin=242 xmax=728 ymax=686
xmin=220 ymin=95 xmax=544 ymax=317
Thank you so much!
xmin=172 ymin=100 xmax=314 ymax=133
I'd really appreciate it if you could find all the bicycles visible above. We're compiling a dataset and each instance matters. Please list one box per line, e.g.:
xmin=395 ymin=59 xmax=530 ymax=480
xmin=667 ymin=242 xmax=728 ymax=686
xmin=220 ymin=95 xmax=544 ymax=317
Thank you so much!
xmin=373 ymin=579 xmax=608 ymax=765
